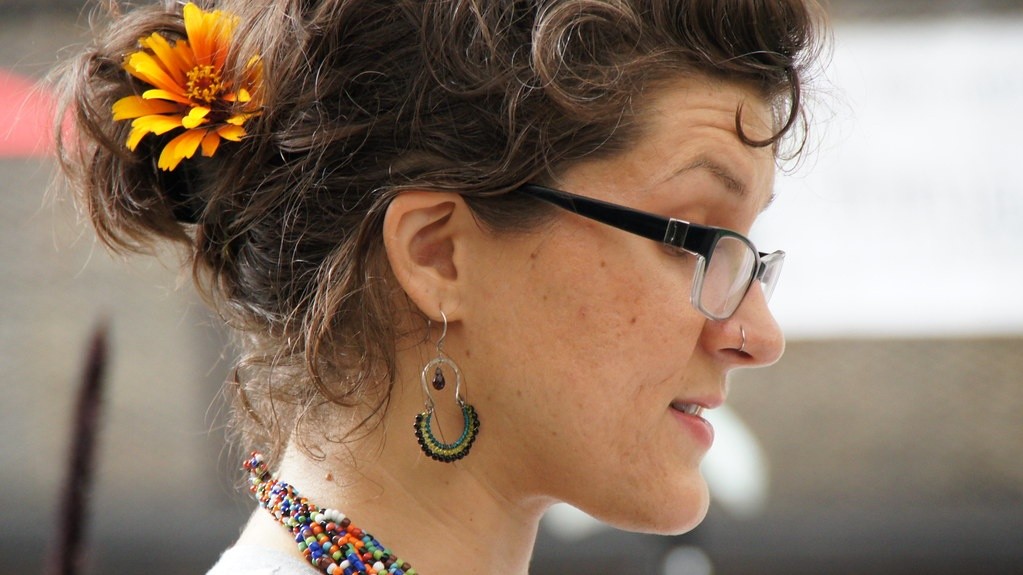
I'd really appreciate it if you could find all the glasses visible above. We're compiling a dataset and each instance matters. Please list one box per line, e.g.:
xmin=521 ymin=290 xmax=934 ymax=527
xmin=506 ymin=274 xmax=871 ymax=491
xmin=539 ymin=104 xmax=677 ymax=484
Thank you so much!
xmin=508 ymin=182 xmax=785 ymax=321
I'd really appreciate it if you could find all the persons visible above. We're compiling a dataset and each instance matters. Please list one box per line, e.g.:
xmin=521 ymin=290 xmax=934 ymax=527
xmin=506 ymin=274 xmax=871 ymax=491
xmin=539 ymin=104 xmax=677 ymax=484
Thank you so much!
xmin=52 ymin=1 xmax=837 ymax=575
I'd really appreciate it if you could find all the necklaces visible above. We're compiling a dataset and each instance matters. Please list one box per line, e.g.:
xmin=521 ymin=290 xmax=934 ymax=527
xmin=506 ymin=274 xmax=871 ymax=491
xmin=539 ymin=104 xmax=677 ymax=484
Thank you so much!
xmin=242 ymin=450 xmax=416 ymax=575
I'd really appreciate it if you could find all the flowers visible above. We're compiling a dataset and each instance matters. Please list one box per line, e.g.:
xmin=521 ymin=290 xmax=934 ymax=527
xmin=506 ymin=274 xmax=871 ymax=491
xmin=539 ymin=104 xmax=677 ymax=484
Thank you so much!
xmin=108 ymin=2 xmax=267 ymax=172
